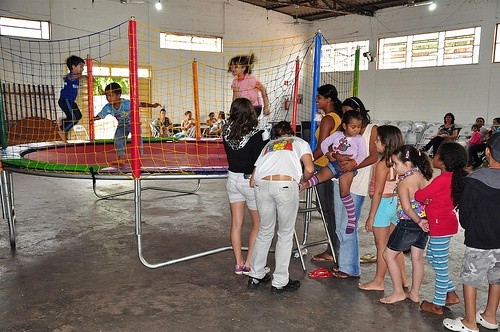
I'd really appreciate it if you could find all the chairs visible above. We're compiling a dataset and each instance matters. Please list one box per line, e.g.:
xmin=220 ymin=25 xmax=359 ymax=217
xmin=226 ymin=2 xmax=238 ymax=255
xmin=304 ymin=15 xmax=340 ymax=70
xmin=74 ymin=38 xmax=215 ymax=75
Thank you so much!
xmin=370 ymin=118 xmax=494 ymax=163
xmin=145 ymin=116 xmax=226 ymax=138
xmin=73 ymin=125 xmax=90 ymax=141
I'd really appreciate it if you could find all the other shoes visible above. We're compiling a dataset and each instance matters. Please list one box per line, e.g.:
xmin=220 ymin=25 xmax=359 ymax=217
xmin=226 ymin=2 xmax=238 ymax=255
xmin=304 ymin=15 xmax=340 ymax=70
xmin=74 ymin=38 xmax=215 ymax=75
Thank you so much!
xmin=419 ymin=148 xmax=425 ymax=153
xmin=428 ymin=155 xmax=434 ymax=159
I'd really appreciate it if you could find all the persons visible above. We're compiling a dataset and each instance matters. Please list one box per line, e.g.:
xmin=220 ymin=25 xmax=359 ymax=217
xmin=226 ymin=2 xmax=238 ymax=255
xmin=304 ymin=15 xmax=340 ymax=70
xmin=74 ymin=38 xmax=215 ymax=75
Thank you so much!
xmin=224 ymin=97 xmax=263 ymax=274
xmin=57 ymin=55 xmax=85 ymax=144
xmin=328 ymin=96 xmax=378 ymax=278
xmin=299 ymin=110 xmax=368 ymax=234
xmin=424 ymin=113 xmax=500 ymax=159
xmin=248 ymin=120 xmax=314 ymax=295
xmin=312 ymin=84 xmax=344 ymax=262
xmin=442 ymin=132 xmax=500 ymax=332
xmin=413 ymin=140 xmax=468 ymax=314
xmin=154 ymin=109 xmax=224 ymax=139
xmin=93 ymin=82 xmax=163 ymax=164
xmin=380 ymin=145 xmax=433 ymax=304
xmin=227 ymin=54 xmax=271 ymax=116
xmin=358 ymin=124 xmax=409 ymax=291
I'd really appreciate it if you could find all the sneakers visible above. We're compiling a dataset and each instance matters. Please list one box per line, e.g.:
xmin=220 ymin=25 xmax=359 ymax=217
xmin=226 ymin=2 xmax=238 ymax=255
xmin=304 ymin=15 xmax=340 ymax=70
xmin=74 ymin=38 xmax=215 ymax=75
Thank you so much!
xmin=235 ymin=264 xmax=245 ymax=275
xmin=272 ymin=279 xmax=301 ymax=293
xmin=243 ymin=264 xmax=271 ymax=275
xmin=248 ymin=275 xmax=272 ymax=289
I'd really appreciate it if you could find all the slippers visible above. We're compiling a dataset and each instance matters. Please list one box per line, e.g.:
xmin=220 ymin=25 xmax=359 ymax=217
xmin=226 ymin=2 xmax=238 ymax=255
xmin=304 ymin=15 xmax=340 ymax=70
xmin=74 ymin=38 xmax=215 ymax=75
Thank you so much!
xmin=443 ymin=317 xmax=479 ymax=332
xmin=312 ymin=252 xmax=333 ymax=262
xmin=332 ymin=271 xmax=361 ymax=278
xmin=309 ymin=267 xmax=331 ymax=278
xmin=333 ymin=264 xmax=340 ymax=270
xmin=476 ymin=310 xmax=499 ymax=329
xmin=360 ymin=253 xmax=377 ymax=263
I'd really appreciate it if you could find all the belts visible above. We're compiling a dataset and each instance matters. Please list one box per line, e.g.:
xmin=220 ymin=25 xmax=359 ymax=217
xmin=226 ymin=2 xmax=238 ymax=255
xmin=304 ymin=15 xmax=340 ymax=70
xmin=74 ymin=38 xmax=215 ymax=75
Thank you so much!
xmin=263 ymin=174 xmax=295 ymax=181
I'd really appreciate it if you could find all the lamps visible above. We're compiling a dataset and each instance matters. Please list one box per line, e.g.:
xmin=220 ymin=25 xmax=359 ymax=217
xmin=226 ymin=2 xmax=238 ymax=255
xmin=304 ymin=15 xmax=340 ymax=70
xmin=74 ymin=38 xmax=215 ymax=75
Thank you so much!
xmin=362 ymin=51 xmax=373 ymax=62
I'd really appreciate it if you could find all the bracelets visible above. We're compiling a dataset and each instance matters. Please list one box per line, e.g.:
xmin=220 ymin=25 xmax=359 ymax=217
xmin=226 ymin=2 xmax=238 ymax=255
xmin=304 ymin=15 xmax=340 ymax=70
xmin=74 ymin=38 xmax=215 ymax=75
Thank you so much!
xmin=418 ymin=218 xmax=421 ymax=223
